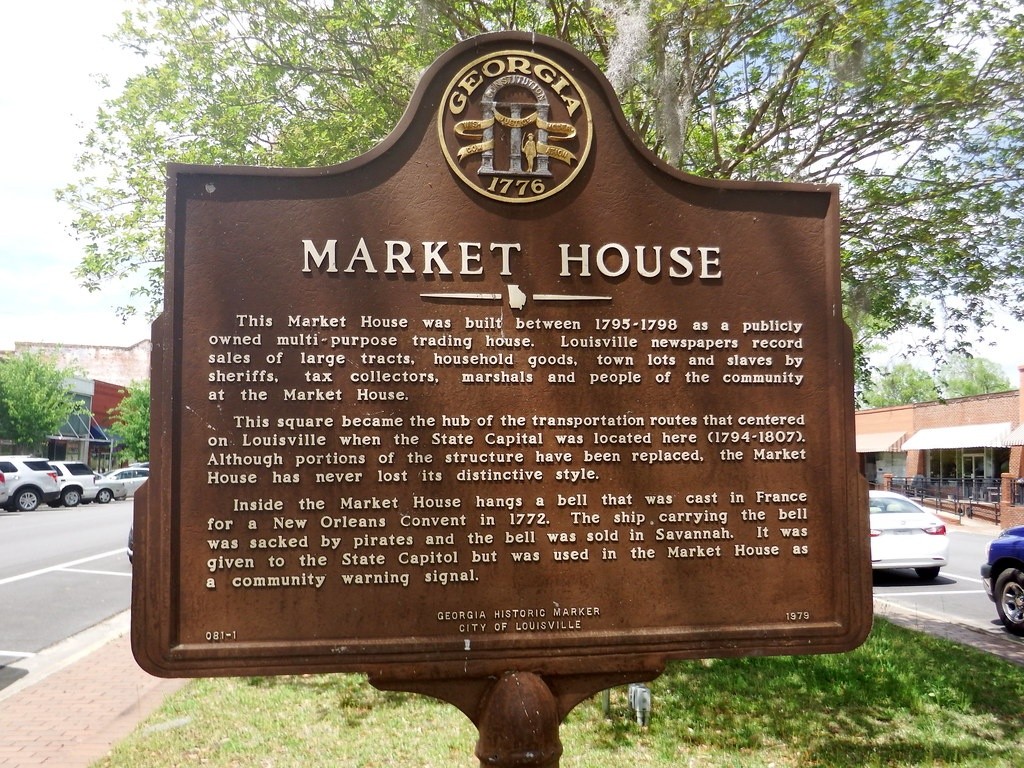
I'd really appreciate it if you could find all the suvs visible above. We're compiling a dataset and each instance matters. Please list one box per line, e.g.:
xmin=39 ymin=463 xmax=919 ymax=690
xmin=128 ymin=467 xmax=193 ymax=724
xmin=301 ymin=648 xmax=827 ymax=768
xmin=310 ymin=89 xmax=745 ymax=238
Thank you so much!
xmin=47 ymin=460 xmax=98 ymax=508
xmin=0 ymin=454 xmax=62 ymax=512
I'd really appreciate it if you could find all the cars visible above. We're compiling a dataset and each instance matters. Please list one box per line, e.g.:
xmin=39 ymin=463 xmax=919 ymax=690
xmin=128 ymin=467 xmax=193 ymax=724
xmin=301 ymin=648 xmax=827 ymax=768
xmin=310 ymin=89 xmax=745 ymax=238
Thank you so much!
xmin=868 ymin=489 xmax=949 ymax=580
xmin=981 ymin=526 xmax=1024 ymax=636
xmin=101 ymin=467 xmax=150 ymax=501
xmin=92 ymin=469 xmax=128 ymax=504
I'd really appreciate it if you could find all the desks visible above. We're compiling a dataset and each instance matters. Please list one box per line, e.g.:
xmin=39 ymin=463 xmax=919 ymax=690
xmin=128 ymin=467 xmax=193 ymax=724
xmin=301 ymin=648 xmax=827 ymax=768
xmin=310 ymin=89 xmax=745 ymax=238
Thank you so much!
xmin=987 ymin=486 xmax=1001 ymax=503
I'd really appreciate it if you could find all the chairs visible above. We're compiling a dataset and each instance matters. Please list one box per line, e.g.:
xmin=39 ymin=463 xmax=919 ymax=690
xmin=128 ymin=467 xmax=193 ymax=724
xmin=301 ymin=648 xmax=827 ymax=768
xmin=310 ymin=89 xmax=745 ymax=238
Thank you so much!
xmin=886 ymin=504 xmax=902 ymax=512
xmin=870 ymin=506 xmax=882 ymax=514
